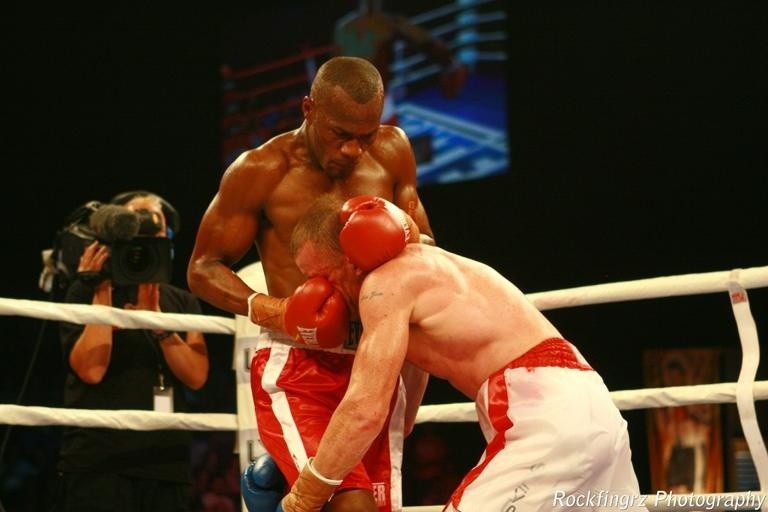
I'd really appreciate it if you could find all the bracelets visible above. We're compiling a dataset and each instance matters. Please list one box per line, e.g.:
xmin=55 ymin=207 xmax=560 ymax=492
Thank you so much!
xmin=150 ymin=330 xmax=179 ymax=341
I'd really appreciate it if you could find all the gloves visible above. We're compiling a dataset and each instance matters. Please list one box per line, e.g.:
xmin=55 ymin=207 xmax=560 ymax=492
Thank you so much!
xmin=248 ymin=275 xmax=350 ymax=349
xmin=241 ymin=452 xmax=344 ymax=512
xmin=340 ymin=195 xmax=419 ymax=272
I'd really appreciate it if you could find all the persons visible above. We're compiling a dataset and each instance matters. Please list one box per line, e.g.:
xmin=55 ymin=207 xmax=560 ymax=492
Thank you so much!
xmin=314 ymin=1 xmax=477 ymax=128
xmin=186 ymin=56 xmax=438 ymax=512
xmin=241 ymin=193 xmax=655 ymax=512
xmin=65 ymin=188 xmax=209 ymax=512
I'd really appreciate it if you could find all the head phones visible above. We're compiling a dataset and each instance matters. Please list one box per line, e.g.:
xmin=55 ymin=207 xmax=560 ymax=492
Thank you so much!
xmin=113 ymin=191 xmax=181 ymax=260
